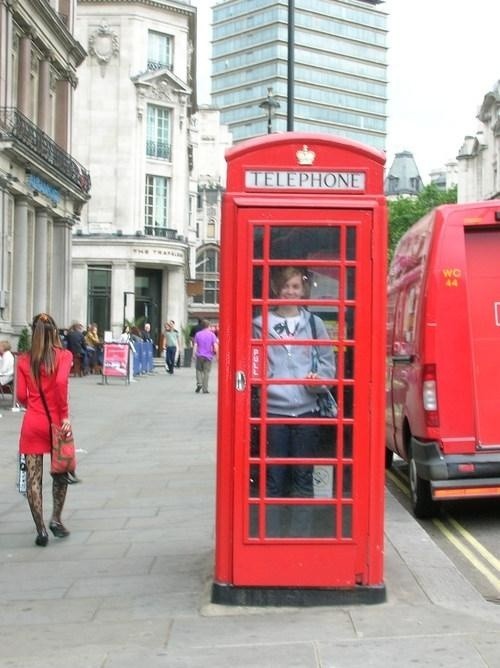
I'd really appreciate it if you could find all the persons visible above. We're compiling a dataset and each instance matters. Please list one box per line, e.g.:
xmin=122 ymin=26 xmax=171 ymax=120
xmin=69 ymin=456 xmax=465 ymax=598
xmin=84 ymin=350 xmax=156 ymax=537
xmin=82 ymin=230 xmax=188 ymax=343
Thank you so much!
xmin=251 ymin=267 xmax=337 ymax=544
xmin=0 ymin=339 xmax=16 ymax=394
xmin=189 ymin=318 xmax=205 ymax=347
xmin=17 ymin=313 xmax=78 ymax=548
xmin=58 ymin=318 xmax=181 ymax=377
xmin=192 ymin=319 xmax=219 ymax=394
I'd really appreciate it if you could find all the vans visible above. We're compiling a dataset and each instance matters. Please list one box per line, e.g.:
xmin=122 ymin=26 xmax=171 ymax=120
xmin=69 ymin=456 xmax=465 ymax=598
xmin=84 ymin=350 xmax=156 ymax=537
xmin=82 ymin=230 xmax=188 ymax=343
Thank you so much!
xmin=380 ymin=198 xmax=500 ymax=526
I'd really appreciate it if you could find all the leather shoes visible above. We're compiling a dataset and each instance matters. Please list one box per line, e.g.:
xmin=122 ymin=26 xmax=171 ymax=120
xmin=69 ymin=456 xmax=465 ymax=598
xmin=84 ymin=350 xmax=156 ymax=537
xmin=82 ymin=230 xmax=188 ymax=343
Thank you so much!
xmin=49 ymin=520 xmax=71 ymax=538
xmin=34 ymin=531 xmax=49 ymax=546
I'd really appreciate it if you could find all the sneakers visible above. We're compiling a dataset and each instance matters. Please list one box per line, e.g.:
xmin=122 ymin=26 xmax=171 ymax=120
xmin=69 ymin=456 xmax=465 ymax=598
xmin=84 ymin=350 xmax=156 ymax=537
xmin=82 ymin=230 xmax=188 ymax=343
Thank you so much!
xmin=195 ymin=385 xmax=210 ymax=395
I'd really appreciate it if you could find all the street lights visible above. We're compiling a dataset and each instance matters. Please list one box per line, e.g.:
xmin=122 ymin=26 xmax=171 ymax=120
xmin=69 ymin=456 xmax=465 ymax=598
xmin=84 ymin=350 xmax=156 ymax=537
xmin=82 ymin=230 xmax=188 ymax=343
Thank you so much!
xmin=259 ymin=86 xmax=281 ymax=148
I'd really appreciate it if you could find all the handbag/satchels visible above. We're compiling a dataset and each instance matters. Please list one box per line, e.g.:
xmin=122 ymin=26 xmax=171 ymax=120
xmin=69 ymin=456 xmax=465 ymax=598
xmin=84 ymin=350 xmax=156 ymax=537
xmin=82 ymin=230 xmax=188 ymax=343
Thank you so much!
xmin=50 ymin=425 xmax=76 ymax=475
xmin=316 ymin=384 xmax=338 ymax=419
xmin=17 ymin=451 xmax=29 ymax=495
